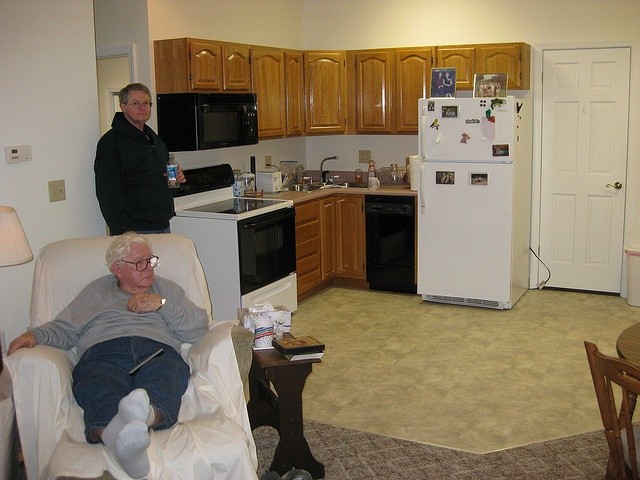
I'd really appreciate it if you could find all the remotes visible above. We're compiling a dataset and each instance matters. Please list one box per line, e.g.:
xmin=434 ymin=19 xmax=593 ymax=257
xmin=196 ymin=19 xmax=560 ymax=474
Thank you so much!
xmin=128 ymin=349 xmax=164 ymax=375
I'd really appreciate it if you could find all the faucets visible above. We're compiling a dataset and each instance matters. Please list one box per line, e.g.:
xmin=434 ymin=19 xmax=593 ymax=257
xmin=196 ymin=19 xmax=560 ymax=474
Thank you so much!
xmin=321 ymin=156 xmax=339 ymax=182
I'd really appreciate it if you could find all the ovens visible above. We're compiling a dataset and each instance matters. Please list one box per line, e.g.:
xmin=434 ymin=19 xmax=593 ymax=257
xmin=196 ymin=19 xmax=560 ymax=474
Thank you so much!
xmin=168 ymin=206 xmax=298 ymax=297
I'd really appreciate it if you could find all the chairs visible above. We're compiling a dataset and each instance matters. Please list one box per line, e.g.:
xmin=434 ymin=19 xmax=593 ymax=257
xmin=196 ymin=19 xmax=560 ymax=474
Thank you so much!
xmin=584 ymin=340 xmax=640 ymax=479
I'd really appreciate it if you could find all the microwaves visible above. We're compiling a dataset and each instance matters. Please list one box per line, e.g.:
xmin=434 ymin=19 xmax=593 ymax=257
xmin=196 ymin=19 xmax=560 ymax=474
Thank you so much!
xmin=157 ymin=92 xmax=259 ymax=151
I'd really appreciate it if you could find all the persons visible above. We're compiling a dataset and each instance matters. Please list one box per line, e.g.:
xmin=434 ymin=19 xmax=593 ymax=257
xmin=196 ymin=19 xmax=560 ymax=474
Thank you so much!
xmin=94 ymin=83 xmax=188 ymax=234
xmin=7 ymin=230 xmax=209 ymax=480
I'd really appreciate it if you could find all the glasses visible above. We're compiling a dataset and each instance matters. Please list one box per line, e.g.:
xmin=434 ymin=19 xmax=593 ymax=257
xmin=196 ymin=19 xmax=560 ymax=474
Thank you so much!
xmin=116 ymin=255 xmax=159 ymax=271
xmin=122 ymin=102 xmax=150 ymax=109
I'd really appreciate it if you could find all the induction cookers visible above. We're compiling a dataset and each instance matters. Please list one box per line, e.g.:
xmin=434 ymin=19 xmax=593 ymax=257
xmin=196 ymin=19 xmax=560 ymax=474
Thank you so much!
xmin=160 ymin=162 xmax=294 ymax=220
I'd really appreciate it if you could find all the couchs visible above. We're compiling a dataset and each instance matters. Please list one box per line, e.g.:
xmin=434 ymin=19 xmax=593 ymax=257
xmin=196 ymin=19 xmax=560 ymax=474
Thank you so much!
xmin=1 ymin=233 xmax=255 ymax=480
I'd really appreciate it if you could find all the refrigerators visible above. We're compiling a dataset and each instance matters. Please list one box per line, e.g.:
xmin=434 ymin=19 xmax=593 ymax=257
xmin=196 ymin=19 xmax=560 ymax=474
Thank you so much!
xmin=417 ymin=97 xmax=533 ymax=311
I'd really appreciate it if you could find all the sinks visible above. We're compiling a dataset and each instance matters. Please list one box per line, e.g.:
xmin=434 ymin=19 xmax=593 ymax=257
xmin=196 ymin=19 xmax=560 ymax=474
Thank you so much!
xmin=338 ymin=182 xmax=368 ymax=187
xmin=286 ymin=185 xmax=321 ymax=191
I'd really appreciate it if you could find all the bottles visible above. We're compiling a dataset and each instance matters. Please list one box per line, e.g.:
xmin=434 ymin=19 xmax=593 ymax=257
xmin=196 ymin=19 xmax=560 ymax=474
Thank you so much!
xmin=368 ymin=158 xmax=376 ymax=178
xmin=391 ymin=163 xmax=398 ymax=181
xmin=166 ymin=153 xmax=180 ymax=189
xmin=295 ymin=164 xmax=307 ymax=184
xmin=355 ymin=166 xmax=362 ymax=185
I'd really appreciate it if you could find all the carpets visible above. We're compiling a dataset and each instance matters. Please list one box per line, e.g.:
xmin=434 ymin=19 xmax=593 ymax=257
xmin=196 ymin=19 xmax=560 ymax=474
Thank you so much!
xmin=250 ymin=415 xmax=640 ymax=480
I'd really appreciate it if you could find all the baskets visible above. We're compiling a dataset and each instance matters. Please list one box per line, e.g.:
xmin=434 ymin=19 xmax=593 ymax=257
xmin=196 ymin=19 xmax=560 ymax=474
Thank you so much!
xmin=377 ymin=167 xmax=406 ymax=184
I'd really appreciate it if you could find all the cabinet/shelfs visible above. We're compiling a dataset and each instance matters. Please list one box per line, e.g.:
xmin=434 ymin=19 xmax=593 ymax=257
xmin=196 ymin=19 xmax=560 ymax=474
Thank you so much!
xmin=335 ymin=196 xmax=363 ymax=276
xmin=153 ymin=36 xmax=252 ymax=93
xmin=294 ymin=201 xmax=321 ymax=295
xmin=347 ymin=45 xmax=432 ymax=135
xmin=250 ymin=45 xmax=305 ymax=141
xmin=303 ymin=50 xmax=348 ymax=136
xmin=434 ymin=42 xmax=530 ymax=91
xmin=320 ymin=197 xmax=337 ymax=281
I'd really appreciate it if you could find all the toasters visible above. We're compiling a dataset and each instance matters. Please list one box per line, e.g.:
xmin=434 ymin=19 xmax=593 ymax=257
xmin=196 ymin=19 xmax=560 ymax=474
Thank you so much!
xmin=256 ymin=170 xmax=283 ymax=193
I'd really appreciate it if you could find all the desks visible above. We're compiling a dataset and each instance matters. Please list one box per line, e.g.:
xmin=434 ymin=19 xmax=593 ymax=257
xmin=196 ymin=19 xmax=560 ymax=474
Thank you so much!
xmin=615 ymin=322 xmax=640 ymax=480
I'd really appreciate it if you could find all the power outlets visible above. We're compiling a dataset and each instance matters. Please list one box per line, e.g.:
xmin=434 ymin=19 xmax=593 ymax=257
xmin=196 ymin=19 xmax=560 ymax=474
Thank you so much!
xmin=358 ymin=150 xmax=372 ymax=163
xmin=265 ymin=156 xmax=272 ymax=169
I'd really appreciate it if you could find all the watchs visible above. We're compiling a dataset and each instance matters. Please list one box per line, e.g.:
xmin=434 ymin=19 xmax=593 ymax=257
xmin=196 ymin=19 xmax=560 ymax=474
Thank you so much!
xmin=158 ymin=295 xmax=168 ymax=312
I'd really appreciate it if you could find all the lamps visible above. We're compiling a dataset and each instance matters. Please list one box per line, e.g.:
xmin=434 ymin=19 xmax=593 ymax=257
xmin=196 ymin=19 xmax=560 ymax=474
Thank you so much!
xmin=0 ymin=205 xmax=35 ymax=377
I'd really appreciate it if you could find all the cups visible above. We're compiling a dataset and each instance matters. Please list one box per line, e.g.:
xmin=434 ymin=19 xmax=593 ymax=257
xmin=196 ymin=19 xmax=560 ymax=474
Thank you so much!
xmin=367 ymin=177 xmax=380 ymax=191
xmin=255 ymin=186 xmax=264 ymax=198
xmin=254 ymin=321 xmax=274 ymax=348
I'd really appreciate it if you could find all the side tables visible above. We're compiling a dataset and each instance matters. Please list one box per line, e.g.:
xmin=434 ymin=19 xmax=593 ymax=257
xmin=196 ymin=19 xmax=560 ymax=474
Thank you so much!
xmin=245 ymin=331 xmax=325 ymax=480
